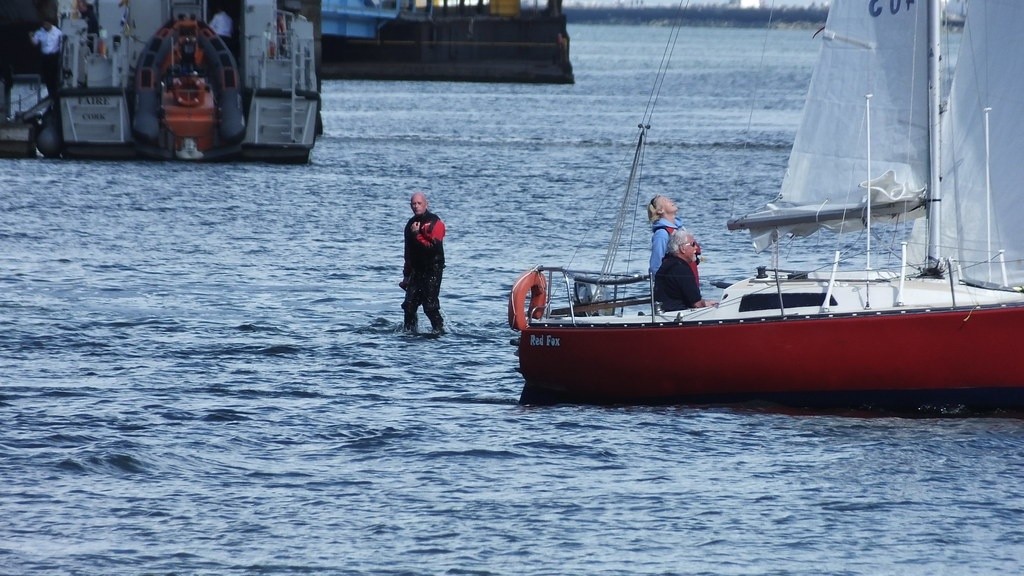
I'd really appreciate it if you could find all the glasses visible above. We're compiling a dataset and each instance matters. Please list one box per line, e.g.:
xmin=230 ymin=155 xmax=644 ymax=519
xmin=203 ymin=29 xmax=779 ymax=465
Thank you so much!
xmin=650 ymin=198 xmax=657 ymax=209
xmin=685 ymin=241 xmax=695 ymax=247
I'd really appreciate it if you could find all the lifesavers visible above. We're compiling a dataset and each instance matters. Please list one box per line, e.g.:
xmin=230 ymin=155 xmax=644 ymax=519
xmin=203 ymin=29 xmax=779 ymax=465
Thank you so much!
xmin=508 ymin=270 xmax=547 ymax=331
xmin=174 ymin=77 xmax=205 ymax=106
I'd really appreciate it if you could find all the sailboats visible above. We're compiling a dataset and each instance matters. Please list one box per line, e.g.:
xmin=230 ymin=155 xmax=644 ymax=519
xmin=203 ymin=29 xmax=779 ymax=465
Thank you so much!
xmin=506 ymin=0 xmax=1024 ymax=406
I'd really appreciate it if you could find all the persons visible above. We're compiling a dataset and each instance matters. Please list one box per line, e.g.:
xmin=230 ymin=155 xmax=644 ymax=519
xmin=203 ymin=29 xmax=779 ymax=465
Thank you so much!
xmin=28 ymin=18 xmax=63 ymax=98
xmin=653 ymin=229 xmax=721 ymax=312
xmin=207 ymin=2 xmax=235 ymax=67
xmin=69 ymin=0 xmax=100 ymax=53
xmin=647 ymin=195 xmax=687 ymax=312
xmin=399 ymin=192 xmax=445 ymax=334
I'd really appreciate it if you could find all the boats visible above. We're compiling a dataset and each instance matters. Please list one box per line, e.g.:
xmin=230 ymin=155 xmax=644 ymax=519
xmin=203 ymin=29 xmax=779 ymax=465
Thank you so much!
xmin=0 ymin=0 xmax=322 ymax=165
xmin=322 ymin=0 xmax=575 ymax=85
